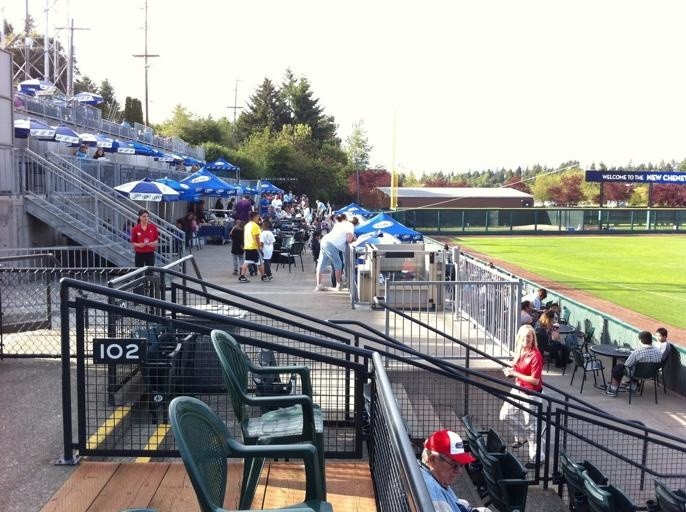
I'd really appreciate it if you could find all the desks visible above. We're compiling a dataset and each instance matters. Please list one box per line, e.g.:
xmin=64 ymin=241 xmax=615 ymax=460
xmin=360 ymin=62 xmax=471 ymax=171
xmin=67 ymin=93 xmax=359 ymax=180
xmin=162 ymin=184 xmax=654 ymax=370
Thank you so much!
xmin=200 ymin=217 xmax=234 ymax=245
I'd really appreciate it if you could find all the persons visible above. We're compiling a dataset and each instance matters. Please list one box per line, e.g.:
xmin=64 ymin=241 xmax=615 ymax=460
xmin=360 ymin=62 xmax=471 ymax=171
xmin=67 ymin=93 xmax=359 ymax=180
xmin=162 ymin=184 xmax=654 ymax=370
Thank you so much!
xmin=102 ymin=191 xmax=359 ymax=306
xmin=601 ymin=330 xmax=662 ymax=397
xmin=415 ymin=429 xmax=494 ymax=512
xmin=442 ymin=243 xmax=574 ymax=367
xmin=498 ymin=323 xmax=546 ymax=467
xmin=624 ymin=327 xmax=671 ymax=387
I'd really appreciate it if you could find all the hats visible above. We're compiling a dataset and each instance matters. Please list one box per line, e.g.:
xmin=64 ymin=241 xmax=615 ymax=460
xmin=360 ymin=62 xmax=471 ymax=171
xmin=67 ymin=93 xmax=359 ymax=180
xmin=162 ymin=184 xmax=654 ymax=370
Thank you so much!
xmin=423 ymin=428 xmax=477 ymax=464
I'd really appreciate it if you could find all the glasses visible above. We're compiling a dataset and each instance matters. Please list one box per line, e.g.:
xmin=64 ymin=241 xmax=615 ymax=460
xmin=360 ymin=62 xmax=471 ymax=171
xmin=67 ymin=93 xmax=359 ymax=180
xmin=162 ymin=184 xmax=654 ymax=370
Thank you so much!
xmin=438 ymin=455 xmax=465 ymax=468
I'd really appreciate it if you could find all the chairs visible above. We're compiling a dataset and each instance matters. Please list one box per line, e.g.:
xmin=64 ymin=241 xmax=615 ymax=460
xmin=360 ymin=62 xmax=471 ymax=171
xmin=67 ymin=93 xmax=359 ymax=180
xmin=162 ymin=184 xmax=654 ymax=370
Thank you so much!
xmin=267 ymin=217 xmax=312 ymax=274
xmin=168 ymin=393 xmax=335 ymax=510
xmin=459 ymin=413 xmax=685 ymax=511
xmin=210 ymin=327 xmax=329 ymax=502
xmin=527 ymin=301 xmax=673 ymax=404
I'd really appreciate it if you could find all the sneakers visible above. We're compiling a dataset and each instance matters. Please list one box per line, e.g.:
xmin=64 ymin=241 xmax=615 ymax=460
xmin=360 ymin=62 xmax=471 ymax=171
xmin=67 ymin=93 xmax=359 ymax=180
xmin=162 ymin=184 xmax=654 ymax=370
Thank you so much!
xmin=525 ymin=459 xmax=545 ymax=469
xmin=511 ymin=440 xmax=528 ymax=448
xmin=232 ymin=268 xmax=274 ymax=283
xmin=600 ymin=379 xmax=640 ymax=397
xmin=315 ymin=282 xmax=346 ymax=292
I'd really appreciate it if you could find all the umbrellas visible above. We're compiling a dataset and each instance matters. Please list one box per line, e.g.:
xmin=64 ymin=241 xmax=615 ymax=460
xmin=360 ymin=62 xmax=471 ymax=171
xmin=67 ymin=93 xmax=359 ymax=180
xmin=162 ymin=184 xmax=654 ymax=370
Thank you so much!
xmin=13 ymin=78 xmax=190 ymax=208
